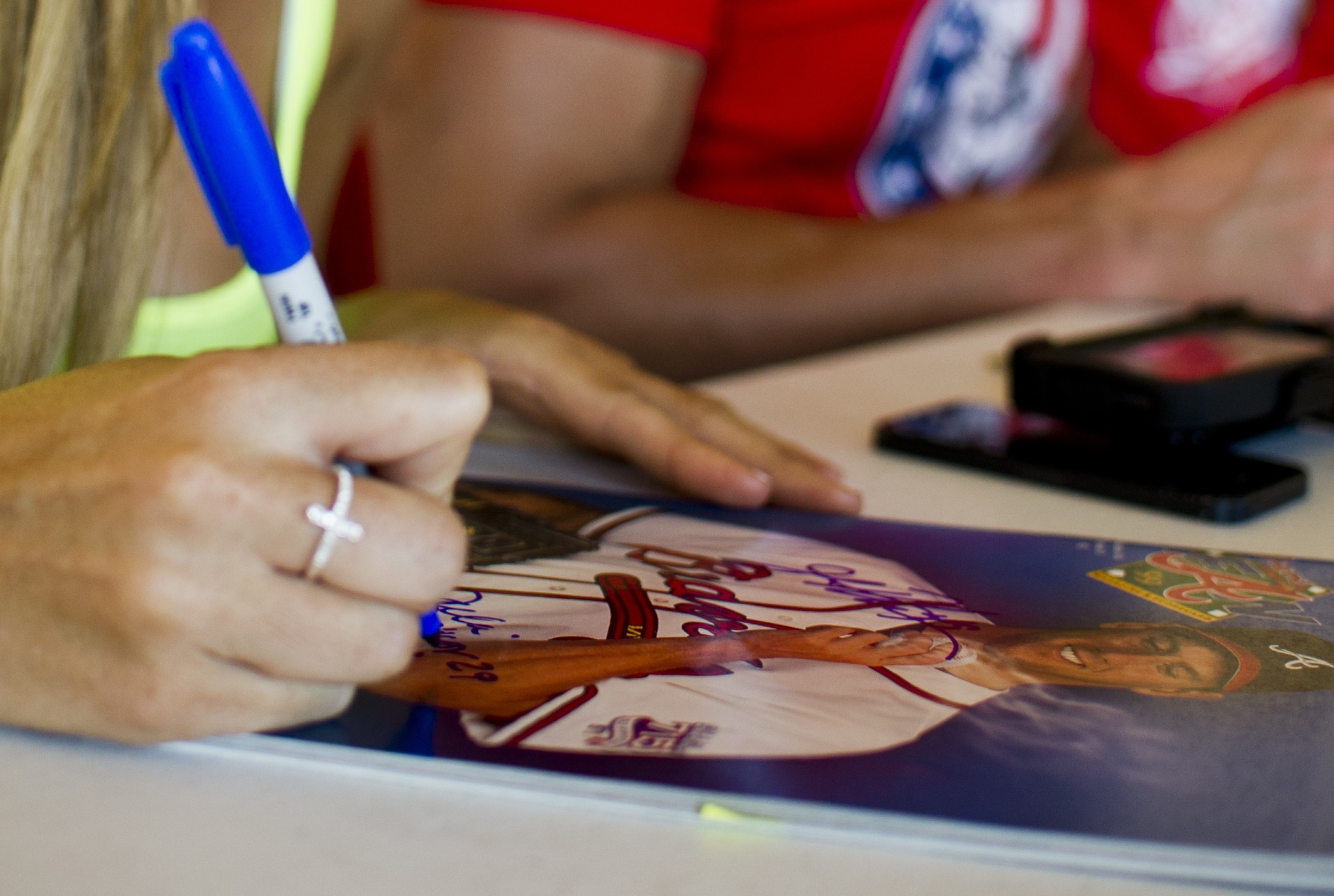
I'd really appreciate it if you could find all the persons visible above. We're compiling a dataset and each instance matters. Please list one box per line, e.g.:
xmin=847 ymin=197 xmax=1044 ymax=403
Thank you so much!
xmin=374 ymin=0 xmax=1334 ymax=384
xmin=0 ymin=2 xmax=864 ymax=750
xmin=361 ymin=481 xmax=1334 ymax=759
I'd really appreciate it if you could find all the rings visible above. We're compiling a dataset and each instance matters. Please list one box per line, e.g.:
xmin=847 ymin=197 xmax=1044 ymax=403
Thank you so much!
xmin=301 ymin=460 xmax=366 ymax=585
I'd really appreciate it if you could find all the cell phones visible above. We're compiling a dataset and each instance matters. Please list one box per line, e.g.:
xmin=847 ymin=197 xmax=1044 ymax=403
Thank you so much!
xmin=1012 ymin=302 xmax=1334 ymax=434
xmin=874 ymin=400 xmax=1310 ymax=524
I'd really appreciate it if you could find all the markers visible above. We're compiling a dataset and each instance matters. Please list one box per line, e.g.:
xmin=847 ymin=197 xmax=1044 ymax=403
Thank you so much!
xmin=154 ymin=16 xmax=448 ymax=652
